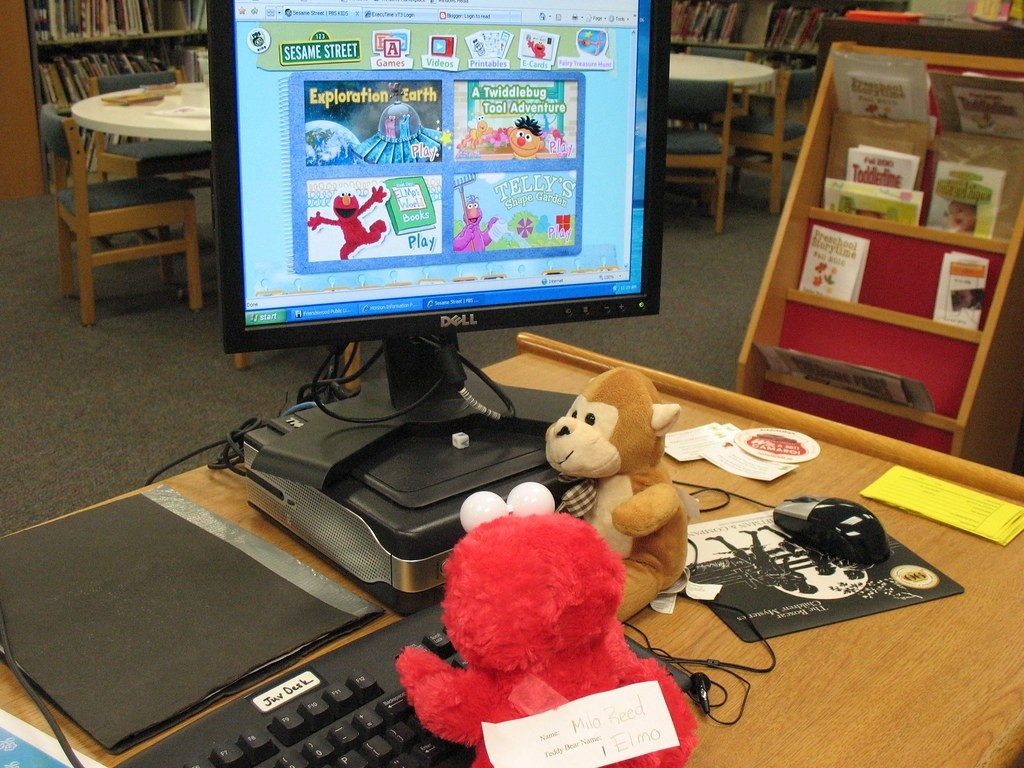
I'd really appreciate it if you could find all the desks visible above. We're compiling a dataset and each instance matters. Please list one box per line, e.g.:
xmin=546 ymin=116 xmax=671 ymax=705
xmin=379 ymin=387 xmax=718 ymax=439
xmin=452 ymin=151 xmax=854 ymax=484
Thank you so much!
xmin=70 ymin=80 xmax=212 ymax=142
xmin=668 ymin=52 xmax=776 ymax=86
xmin=0 ymin=331 xmax=1024 ymax=768
xmin=737 ymin=42 xmax=1024 ymax=476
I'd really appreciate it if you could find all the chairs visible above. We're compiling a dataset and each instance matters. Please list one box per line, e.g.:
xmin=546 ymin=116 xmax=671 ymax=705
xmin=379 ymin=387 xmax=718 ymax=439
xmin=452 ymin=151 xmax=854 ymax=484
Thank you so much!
xmin=664 ymin=62 xmax=817 ymax=233
xmin=39 ymin=70 xmax=213 ymax=327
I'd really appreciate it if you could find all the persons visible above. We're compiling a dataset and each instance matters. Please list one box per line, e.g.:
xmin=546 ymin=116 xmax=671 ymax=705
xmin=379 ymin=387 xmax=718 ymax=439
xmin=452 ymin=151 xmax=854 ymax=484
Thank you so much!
xmin=955 ymin=291 xmax=981 ymax=310
xmin=948 ymin=202 xmax=977 ymax=233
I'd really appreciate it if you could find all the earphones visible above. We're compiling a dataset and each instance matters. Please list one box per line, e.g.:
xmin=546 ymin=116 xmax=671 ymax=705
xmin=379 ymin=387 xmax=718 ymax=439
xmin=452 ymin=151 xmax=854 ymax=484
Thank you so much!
xmin=687 ymin=672 xmax=711 ymax=715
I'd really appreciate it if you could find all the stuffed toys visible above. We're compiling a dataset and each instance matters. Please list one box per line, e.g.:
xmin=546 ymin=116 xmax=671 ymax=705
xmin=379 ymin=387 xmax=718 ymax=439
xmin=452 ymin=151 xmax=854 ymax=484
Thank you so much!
xmin=546 ymin=367 xmax=687 ymax=622
xmin=396 ymin=482 xmax=700 ymax=768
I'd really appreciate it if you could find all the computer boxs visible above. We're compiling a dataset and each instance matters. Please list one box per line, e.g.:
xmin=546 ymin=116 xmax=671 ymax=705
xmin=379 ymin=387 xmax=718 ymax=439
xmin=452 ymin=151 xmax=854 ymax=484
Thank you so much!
xmin=242 ymin=402 xmax=582 ymax=615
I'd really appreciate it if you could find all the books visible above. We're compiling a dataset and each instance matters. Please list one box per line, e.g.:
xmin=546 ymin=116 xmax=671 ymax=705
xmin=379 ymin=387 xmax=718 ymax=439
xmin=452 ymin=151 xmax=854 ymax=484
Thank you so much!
xmin=823 ymin=144 xmax=924 ymax=226
xmin=799 ymin=225 xmax=870 ymax=303
xmin=670 ymin=0 xmax=839 ymax=95
xmin=33 ymin=0 xmax=210 ymax=175
xmin=926 ymin=160 xmax=1006 ymax=238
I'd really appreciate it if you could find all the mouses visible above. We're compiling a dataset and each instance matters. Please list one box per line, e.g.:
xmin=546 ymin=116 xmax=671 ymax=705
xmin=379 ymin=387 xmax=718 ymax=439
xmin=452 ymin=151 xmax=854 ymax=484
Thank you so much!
xmin=773 ymin=494 xmax=891 ymax=564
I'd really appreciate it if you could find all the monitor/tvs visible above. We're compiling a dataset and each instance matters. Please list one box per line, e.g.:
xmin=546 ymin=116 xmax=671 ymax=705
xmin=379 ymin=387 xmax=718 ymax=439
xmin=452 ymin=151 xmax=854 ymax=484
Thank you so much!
xmin=203 ymin=1 xmax=672 ymax=489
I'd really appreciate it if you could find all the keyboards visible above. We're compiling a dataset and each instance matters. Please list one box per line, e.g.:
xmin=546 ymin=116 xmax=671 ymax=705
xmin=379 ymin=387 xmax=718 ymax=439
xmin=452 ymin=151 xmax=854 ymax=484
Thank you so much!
xmin=113 ymin=596 xmax=694 ymax=768
